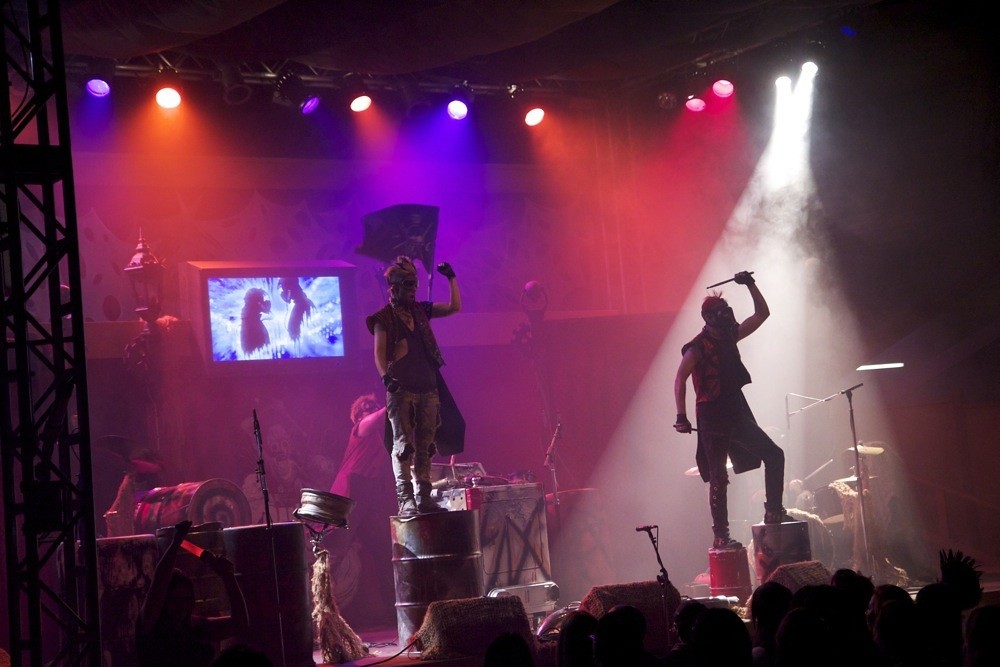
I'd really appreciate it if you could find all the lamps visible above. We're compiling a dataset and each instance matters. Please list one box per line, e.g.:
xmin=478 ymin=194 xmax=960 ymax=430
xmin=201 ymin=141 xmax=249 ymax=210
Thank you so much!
xmin=658 ymin=38 xmax=831 ymax=111
xmin=83 ymin=57 xmax=544 ymax=128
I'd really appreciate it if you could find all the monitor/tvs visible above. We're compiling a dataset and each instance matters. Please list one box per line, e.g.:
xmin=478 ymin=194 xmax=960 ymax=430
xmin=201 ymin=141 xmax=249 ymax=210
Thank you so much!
xmin=206 ymin=276 xmax=345 ymax=362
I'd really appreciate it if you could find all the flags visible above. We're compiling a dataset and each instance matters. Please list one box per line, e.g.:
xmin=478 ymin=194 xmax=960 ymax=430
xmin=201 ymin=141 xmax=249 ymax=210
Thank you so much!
xmin=356 ymin=203 xmax=442 ymax=275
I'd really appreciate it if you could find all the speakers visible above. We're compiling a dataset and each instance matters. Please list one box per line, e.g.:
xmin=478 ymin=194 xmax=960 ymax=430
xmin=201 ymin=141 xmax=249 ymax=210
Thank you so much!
xmin=419 ymin=595 xmax=535 ymax=660
xmin=580 ymin=578 xmax=681 ymax=651
xmin=745 ymin=559 xmax=834 ymax=621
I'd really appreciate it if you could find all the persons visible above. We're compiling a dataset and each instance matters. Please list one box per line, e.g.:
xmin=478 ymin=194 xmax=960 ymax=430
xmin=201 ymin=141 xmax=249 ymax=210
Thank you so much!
xmin=315 ymin=394 xmax=396 ymax=619
xmin=362 ymin=258 xmax=467 ymax=521
xmin=560 ymin=567 xmax=999 ymax=667
xmin=674 ymin=270 xmax=796 ymax=550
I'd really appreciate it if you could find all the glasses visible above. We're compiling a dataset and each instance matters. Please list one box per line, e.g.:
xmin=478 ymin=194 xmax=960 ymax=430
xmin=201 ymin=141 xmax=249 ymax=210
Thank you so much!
xmin=402 ymin=279 xmax=418 ymax=289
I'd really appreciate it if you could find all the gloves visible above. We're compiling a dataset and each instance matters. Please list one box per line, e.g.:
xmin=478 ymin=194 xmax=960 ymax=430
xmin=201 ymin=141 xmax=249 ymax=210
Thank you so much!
xmin=437 ymin=262 xmax=456 ymax=279
xmin=734 ymin=271 xmax=755 ymax=286
xmin=381 ymin=374 xmax=401 ymax=393
xmin=676 ymin=414 xmax=691 ymax=434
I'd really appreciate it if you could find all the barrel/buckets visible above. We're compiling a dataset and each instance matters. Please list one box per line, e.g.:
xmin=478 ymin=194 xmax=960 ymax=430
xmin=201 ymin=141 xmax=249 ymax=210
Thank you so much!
xmin=752 ymin=520 xmax=812 ymax=588
xmin=222 ymin=522 xmax=313 ymax=667
xmin=710 ymin=546 xmax=754 ymax=608
xmin=155 ymin=520 xmax=242 ymax=658
xmin=57 ymin=534 xmax=159 ymax=667
xmin=389 ymin=509 xmax=486 ymax=653
xmin=130 ymin=478 xmax=253 ymax=535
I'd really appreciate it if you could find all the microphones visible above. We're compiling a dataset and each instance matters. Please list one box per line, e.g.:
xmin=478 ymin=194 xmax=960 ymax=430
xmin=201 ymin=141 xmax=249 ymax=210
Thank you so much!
xmin=785 ymin=394 xmax=790 ymax=430
xmin=635 ymin=525 xmax=657 ymax=532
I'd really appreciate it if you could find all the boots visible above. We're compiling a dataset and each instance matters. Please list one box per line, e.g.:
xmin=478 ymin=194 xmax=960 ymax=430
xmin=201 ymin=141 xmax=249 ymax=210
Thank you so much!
xmin=414 ymin=481 xmax=448 ymax=513
xmin=712 ymin=526 xmax=742 ymax=548
xmin=397 ymin=483 xmax=417 ymax=515
xmin=764 ymin=501 xmax=794 ymax=524
